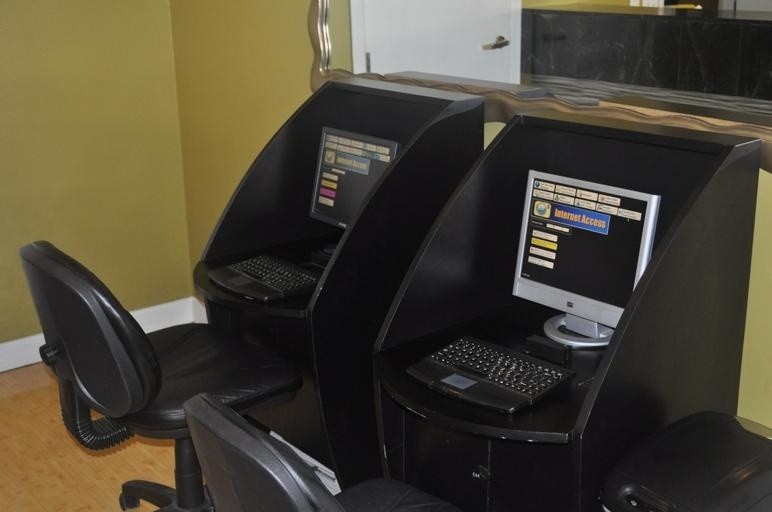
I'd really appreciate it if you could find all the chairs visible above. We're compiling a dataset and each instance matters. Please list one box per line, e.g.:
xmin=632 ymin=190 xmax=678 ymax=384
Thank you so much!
xmin=179 ymin=389 xmax=465 ymax=510
xmin=17 ymin=239 xmax=307 ymax=512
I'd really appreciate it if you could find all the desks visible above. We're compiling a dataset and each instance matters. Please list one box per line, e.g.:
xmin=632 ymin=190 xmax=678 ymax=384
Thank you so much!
xmin=374 ymin=305 xmax=745 ymax=511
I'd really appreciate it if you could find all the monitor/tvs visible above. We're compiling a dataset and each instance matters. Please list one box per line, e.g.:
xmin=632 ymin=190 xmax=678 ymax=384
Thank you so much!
xmin=512 ymin=169 xmax=662 ymax=351
xmin=309 ymin=125 xmax=401 ymax=257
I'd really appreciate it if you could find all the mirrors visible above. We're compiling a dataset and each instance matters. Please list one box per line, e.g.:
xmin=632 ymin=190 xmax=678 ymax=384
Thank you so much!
xmin=306 ymin=3 xmax=771 ymax=107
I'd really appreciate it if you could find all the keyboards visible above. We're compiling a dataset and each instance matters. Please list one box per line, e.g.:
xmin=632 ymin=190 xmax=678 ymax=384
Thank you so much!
xmin=406 ymin=335 xmax=574 ymax=415
xmin=207 ymin=254 xmax=321 ymax=303
